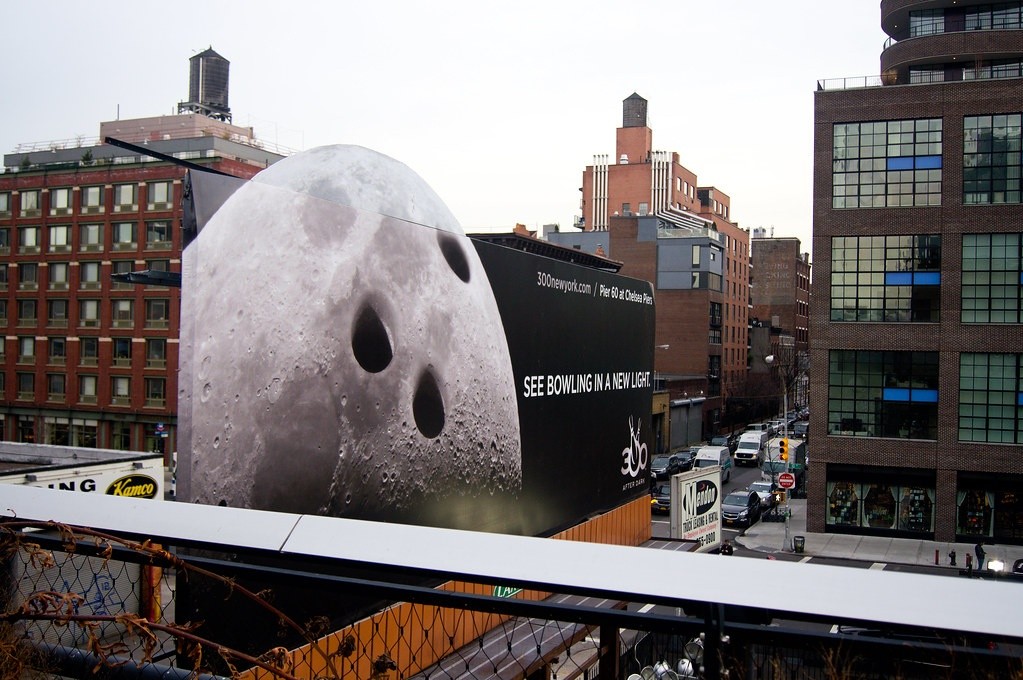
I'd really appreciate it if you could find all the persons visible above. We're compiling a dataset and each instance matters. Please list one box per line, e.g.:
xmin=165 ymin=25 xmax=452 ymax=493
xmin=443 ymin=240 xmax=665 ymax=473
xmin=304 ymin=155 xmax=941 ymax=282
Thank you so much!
xmin=975 ymin=540 xmax=987 ymax=574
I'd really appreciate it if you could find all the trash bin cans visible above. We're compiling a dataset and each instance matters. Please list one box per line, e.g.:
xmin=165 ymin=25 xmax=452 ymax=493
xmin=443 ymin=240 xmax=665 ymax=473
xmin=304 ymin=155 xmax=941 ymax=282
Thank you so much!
xmin=794 ymin=536 xmax=805 ymax=552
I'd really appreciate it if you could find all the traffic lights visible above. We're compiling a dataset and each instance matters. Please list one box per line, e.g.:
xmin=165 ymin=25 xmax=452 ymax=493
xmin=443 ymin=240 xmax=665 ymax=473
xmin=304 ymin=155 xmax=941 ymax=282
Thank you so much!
xmin=779 ymin=438 xmax=788 ymax=460
xmin=772 ymin=492 xmax=782 ymax=502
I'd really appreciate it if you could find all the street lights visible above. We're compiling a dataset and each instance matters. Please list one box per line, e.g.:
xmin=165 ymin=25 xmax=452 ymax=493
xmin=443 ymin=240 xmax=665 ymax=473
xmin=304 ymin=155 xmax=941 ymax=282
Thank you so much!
xmin=766 ymin=354 xmax=792 ymax=551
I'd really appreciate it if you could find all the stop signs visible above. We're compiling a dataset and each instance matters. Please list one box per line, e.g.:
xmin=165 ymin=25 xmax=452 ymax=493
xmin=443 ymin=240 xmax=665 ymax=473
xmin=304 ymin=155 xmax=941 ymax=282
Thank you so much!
xmin=779 ymin=473 xmax=794 ymax=488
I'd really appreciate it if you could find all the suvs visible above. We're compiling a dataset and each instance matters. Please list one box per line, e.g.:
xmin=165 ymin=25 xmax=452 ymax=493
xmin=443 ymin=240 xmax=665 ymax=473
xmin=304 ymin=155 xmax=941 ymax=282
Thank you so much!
xmin=721 ymin=488 xmax=761 ymax=528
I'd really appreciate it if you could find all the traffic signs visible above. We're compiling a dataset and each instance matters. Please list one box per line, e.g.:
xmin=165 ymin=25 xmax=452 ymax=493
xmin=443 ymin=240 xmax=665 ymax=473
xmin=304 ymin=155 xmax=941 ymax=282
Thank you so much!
xmin=777 ymin=430 xmax=795 ymax=436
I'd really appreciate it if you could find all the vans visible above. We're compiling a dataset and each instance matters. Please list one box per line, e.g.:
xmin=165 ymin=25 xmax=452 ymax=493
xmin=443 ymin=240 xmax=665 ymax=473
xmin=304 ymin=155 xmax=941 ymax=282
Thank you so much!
xmin=691 ymin=446 xmax=731 ymax=485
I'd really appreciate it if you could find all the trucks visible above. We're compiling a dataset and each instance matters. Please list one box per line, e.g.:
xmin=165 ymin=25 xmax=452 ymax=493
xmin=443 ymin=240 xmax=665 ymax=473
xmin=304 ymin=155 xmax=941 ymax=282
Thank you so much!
xmin=734 ymin=432 xmax=768 ymax=468
xmin=761 ymin=439 xmax=806 ymax=491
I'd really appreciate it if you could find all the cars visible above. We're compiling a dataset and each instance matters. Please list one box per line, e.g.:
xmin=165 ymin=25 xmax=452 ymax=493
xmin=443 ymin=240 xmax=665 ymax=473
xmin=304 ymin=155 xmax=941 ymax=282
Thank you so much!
xmin=651 ymin=471 xmax=656 ymax=492
xmin=651 ymin=484 xmax=671 ymax=515
xmin=744 ymin=406 xmax=810 ymax=440
xmin=651 ymin=454 xmax=681 ymax=481
xmin=833 ymin=626 xmax=998 ymax=680
xmin=674 ymin=450 xmax=698 ymax=472
xmin=746 ymin=481 xmax=778 ymax=510
xmin=707 ymin=435 xmax=737 ymax=456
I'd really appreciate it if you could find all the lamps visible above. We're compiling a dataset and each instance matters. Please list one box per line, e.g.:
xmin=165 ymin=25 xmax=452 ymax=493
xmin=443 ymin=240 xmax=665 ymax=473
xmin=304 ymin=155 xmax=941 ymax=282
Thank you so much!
xmin=25 ymin=475 xmax=37 ymax=482
xmin=132 ymin=463 xmax=142 ymax=469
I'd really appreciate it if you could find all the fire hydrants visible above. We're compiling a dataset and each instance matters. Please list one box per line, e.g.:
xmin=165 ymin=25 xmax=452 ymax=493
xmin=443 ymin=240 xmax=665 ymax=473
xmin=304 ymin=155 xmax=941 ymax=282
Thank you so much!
xmin=949 ymin=549 xmax=957 ymax=566
